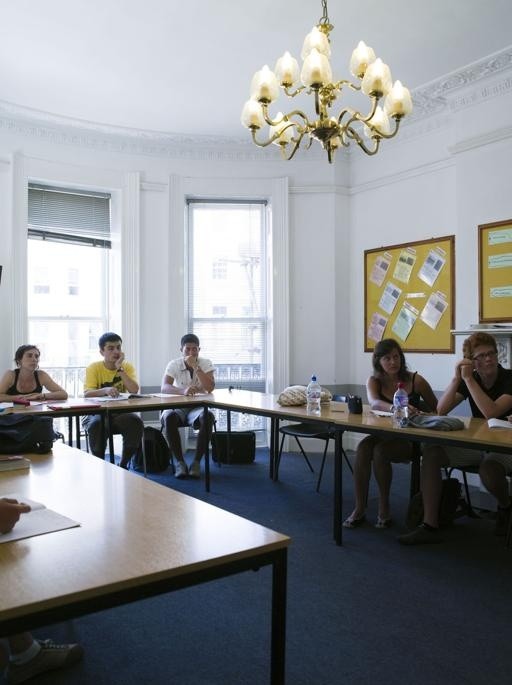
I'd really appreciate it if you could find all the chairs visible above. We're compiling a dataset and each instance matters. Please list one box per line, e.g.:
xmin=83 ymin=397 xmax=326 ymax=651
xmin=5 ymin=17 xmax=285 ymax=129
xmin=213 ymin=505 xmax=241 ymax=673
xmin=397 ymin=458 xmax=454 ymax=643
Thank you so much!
xmin=274 ymin=394 xmax=354 ymax=490
xmin=76 ymin=430 xmax=91 ymax=453
xmin=446 ymin=467 xmax=512 ymax=510
xmin=158 ymin=411 xmax=221 ymax=474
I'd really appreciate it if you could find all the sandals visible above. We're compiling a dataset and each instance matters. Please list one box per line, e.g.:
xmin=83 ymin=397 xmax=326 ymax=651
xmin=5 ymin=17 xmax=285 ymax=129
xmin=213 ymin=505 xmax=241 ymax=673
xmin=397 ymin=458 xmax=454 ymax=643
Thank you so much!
xmin=342 ymin=506 xmax=369 ymax=529
xmin=373 ymin=503 xmax=393 ymax=530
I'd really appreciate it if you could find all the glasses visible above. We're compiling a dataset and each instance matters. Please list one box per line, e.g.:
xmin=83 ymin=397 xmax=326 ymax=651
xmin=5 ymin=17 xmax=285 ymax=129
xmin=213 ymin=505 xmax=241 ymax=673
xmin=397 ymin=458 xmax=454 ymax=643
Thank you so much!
xmin=470 ymin=350 xmax=498 ymax=361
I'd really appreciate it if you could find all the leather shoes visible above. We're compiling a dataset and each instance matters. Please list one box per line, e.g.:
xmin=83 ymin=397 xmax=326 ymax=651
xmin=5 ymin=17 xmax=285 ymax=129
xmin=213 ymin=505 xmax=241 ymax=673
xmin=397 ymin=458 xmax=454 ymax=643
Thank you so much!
xmin=393 ymin=523 xmax=446 ymax=547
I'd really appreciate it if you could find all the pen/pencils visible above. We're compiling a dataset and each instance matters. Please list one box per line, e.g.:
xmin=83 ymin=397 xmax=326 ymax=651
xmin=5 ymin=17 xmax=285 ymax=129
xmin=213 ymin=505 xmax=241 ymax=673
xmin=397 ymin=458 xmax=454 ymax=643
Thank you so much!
xmin=330 ymin=410 xmax=345 ymax=413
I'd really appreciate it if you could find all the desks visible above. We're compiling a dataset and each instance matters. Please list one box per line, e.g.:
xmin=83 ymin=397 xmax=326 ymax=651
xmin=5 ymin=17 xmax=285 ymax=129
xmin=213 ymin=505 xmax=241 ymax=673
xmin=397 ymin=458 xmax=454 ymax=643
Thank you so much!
xmin=206 ymin=392 xmax=379 ymax=540
xmin=0 ymin=397 xmax=109 ymax=460
xmin=0 ymin=441 xmax=291 ymax=685
xmin=336 ymin=412 xmax=512 ymax=546
xmin=77 ymin=389 xmax=276 ymax=493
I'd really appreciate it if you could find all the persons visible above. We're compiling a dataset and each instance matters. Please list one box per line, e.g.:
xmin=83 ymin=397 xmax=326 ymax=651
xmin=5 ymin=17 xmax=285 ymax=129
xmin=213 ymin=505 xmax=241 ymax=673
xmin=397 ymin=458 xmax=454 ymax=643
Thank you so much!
xmin=0 ymin=344 xmax=68 ymax=405
xmin=394 ymin=331 xmax=512 ymax=545
xmin=342 ymin=339 xmax=438 ymax=529
xmin=82 ymin=332 xmax=144 ymax=469
xmin=0 ymin=499 xmax=87 ymax=684
xmin=160 ymin=333 xmax=215 ymax=479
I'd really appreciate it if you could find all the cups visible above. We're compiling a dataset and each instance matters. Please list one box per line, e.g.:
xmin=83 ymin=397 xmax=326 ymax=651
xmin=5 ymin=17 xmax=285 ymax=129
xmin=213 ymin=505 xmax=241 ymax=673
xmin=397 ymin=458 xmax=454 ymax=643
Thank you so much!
xmin=347 ymin=397 xmax=362 ymax=414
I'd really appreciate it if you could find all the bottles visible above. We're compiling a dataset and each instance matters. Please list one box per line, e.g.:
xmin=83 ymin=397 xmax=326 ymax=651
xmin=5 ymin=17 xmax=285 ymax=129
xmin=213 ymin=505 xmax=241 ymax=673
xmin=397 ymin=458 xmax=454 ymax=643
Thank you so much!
xmin=307 ymin=375 xmax=321 ymax=415
xmin=393 ymin=383 xmax=408 ymax=427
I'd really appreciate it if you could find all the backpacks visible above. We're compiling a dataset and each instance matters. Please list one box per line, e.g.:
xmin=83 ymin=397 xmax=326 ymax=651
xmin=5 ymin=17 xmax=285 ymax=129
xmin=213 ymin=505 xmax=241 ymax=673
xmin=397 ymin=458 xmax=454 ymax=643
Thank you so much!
xmin=131 ymin=426 xmax=171 ymax=474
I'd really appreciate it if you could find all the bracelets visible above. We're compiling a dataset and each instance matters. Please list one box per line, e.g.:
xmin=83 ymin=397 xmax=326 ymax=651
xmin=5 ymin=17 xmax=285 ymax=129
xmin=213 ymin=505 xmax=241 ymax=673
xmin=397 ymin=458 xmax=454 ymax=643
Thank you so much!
xmin=39 ymin=392 xmax=46 ymax=401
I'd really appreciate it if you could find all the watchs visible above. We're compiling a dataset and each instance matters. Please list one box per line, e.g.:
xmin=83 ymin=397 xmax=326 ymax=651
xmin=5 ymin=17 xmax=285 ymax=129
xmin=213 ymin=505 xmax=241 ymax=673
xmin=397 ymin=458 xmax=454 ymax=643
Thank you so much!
xmin=117 ymin=366 xmax=124 ymax=373
xmin=192 ymin=365 xmax=200 ymax=372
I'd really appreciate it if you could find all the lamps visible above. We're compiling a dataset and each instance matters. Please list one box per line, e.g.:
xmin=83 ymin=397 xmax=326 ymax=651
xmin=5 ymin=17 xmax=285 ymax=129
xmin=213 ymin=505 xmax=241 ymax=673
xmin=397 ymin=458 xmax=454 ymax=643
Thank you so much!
xmin=240 ymin=0 xmax=412 ymax=164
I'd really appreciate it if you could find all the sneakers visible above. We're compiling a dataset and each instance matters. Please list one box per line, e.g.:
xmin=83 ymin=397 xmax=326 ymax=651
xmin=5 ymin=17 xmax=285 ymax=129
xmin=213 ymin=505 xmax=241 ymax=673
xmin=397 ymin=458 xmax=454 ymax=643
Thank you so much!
xmin=2 ymin=639 xmax=85 ymax=685
xmin=173 ymin=460 xmax=190 ymax=478
xmin=188 ymin=459 xmax=202 ymax=478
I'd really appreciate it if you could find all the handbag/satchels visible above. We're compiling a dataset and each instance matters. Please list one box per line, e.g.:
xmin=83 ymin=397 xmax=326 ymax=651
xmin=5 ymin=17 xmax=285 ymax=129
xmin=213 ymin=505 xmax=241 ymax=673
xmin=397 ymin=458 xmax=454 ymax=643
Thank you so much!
xmin=210 ymin=430 xmax=256 ymax=466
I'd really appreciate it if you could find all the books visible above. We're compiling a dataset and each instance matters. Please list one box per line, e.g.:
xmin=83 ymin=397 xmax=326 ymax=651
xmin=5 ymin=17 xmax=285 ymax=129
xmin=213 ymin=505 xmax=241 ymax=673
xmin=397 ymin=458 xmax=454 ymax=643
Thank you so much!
xmin=0 ymin=399 xmax=66 ymax=415
xmin=370 ymin=409 xmax=394 ymax=417
xmin=1 ymin=495 xmax=81 ymax=544
xmin=0 ymin=455 xmax=31 ymax=473
xmin=487 ymin=418 xmax=512 ymax=428
xmin=85 ymin=392 xmax=151 ymax=401
